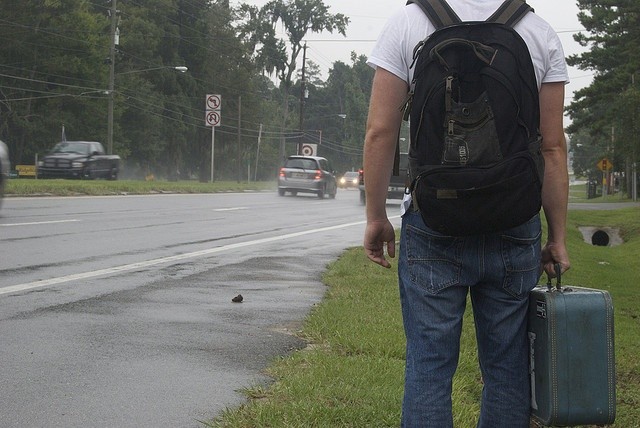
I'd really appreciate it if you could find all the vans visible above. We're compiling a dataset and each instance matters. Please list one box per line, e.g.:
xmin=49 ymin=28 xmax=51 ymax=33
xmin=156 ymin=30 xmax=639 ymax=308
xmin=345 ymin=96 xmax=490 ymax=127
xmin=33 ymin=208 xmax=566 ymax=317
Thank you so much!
xmin=360 ymin=152 xmax=413 ymax=204
xmin=277 ymin=155 xmax=337 ymax=199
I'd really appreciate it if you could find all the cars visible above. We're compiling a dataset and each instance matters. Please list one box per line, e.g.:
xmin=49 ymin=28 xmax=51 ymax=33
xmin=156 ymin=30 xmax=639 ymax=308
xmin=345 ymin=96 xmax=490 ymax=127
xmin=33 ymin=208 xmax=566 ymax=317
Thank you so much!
xmin=339 ymin=170 xmax=360 ymax=188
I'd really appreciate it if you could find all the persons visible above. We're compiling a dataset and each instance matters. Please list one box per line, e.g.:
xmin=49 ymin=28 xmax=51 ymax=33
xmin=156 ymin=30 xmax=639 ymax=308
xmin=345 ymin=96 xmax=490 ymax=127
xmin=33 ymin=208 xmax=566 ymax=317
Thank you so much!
xmin=363 ymin=0 xmax=570 ymax=427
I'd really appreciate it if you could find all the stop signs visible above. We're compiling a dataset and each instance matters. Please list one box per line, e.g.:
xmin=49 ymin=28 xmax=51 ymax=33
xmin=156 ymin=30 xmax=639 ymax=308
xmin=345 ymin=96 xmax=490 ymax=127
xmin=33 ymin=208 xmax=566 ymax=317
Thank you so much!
xmin=205 ymin=93 xmax=221 ymax=110
xmin=205 ymin=110 xmax=221 ymax=127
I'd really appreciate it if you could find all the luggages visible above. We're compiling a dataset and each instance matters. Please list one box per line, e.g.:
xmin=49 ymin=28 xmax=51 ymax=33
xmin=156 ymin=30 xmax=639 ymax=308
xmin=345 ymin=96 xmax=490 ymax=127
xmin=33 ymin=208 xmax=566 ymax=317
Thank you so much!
xmin=529 ymin=261 xmax=616 ymax=426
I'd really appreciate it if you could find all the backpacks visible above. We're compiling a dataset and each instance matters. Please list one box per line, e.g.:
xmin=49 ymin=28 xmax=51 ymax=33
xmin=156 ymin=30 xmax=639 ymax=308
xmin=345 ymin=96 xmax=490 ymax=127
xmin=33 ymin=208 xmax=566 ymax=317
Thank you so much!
xmin=395 ymin=0 xmax=546 ymax=236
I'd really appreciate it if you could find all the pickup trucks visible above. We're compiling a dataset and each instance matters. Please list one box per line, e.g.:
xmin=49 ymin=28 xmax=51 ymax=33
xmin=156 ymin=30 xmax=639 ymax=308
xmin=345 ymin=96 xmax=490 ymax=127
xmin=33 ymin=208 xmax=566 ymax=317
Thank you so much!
xmin=36 ymin=140 xmax=121 ymax=180
